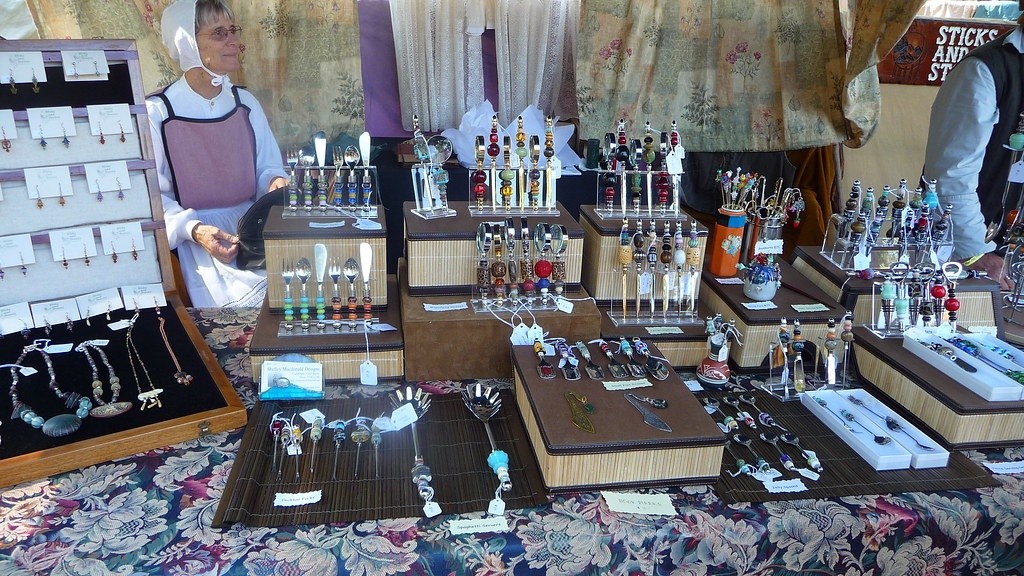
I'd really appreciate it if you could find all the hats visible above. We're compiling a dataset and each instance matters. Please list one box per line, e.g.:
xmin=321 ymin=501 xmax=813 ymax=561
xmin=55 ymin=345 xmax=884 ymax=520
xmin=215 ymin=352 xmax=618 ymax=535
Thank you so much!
xmin=161 ymin=1 xmax=235 ymax=97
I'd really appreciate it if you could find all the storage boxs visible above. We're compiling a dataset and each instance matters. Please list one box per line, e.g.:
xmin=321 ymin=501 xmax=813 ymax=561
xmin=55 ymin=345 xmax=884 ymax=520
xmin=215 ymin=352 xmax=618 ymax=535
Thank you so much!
xmin=263 ymin=204 xmax=389 ymax=312
xmin=699 ymin=254 xmax=854 ymax=372
xmin=849 ymin=325 xmax=1024 ymax=447
xmin=0 ymin=38 xmax=248 ymax=488
xmin=597 ymin=306 xmax=732 ymax=369
xmin=578 ymin=205 xmax=709 ymax=308
xmin=511 ymin=340 xmax=727 ymax=490
xmin=402 ymin=201 xmax=584 ymax=297
xmin=249 ymin=273 xmax=405 ymax=383
xmin=397 ymin=257 xmax=602 ymax=381
xmin=787 ymin=245 xmax=1005 ymax=342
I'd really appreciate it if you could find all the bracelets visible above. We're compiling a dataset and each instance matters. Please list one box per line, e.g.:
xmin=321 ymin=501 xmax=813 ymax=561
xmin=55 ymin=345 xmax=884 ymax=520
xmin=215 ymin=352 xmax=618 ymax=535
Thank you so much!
xmin=960 ymin=254 xmax=991 ymax=267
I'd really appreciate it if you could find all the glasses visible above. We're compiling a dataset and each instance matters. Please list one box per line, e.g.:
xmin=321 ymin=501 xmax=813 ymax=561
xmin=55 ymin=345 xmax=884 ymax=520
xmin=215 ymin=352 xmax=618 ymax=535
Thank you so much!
xmin=196 ymin=24 xmax=243 ymax=41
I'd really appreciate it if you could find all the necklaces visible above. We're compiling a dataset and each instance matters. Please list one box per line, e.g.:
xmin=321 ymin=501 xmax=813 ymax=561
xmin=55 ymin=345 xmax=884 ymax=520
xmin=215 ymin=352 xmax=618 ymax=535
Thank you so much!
xmin=76 ymin=340 xmax=134 ymax=418
xmin=10 ymin=339 xmax=92 ymax=437
xmin=158 ymin=316 xmax=193 ymax=385
xmin=121 ymin=313 xmax=165 ymax=411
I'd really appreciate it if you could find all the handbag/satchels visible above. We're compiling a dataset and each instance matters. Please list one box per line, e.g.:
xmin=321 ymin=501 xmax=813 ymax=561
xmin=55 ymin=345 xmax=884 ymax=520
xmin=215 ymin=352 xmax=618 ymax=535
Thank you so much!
xmin=233 ymin=179 xmax=319 ymax=271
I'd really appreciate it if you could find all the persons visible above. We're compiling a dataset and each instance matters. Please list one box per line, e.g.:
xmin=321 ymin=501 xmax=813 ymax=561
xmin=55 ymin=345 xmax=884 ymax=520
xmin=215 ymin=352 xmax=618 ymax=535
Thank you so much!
xmin=912 ymin=12 xmax=1024 ymax=291
xmin=142 ymin=0 xmax=294 ymax=308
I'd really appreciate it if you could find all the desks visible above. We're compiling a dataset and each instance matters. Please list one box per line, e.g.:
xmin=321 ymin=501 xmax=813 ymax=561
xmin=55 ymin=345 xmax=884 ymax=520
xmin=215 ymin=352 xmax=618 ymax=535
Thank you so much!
xmin=0 ymin=309 xmax=1024 ymax=576
